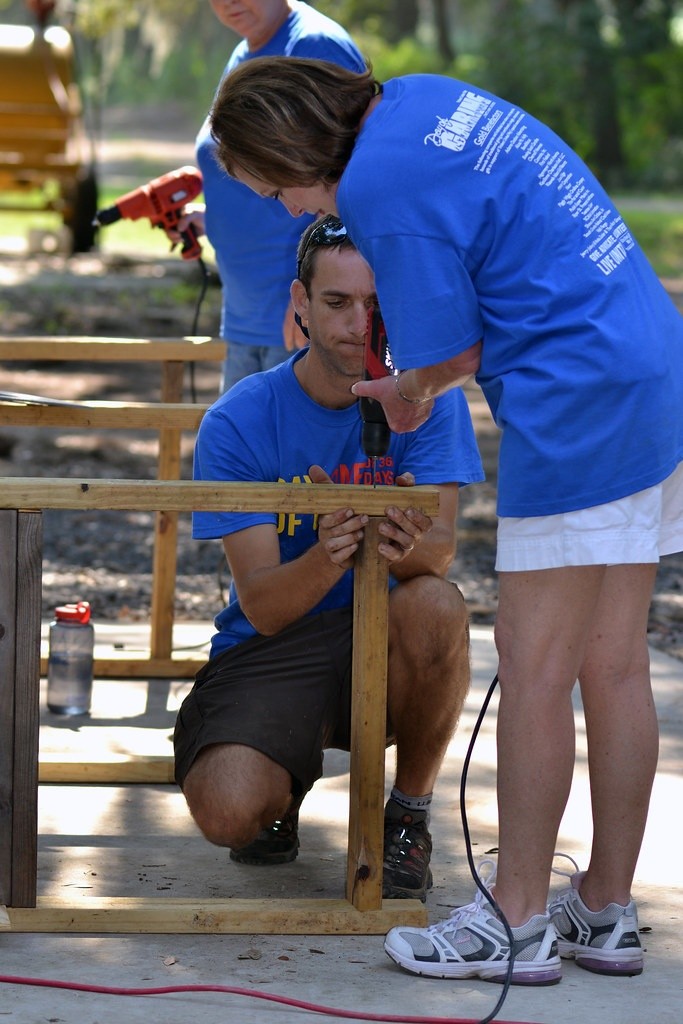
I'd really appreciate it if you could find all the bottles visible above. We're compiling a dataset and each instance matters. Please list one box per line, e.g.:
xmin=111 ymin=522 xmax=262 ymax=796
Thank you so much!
xmin=48 ymin=602 xmax=93 ymax=715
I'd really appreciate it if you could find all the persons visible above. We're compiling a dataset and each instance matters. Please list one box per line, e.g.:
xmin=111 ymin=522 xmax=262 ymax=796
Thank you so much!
xmin=213 ymin=57 xmax=683 ymax=983
xmin=175 ymin=217 xmax=471 ymax=898
xmin=195 ymin=0 xmax=368 ymax=628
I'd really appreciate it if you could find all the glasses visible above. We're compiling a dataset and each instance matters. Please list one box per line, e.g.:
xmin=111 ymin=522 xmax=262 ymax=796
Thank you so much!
xmin=297 ymin=220 xmax=348 ymax=279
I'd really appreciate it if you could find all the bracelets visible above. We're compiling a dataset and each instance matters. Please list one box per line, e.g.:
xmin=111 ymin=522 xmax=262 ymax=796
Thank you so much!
xmin=396 ymin=371 xmax=432 ymax=402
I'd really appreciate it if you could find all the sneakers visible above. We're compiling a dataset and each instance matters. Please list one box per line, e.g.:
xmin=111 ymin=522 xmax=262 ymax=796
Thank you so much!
xmin=381 ymin=799 xmax=433 ymax=903
xmin=228 ymin=785 xmax=312 ymax=865
xmin=382 ymin=860 xmax=563 ymax=985
xmin=545 ymin=853 xmax=644 ymax=976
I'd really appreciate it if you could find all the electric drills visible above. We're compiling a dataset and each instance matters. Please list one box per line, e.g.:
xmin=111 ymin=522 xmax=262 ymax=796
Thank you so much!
xmin=359 ymin=298 xmax=405 ymax=488
xmin=83 ymin=165 xmax=204 ymax=261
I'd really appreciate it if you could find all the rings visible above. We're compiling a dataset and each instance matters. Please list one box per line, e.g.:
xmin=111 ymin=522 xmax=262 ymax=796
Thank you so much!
xmin=401 ymin=544 xmax=414 ymax=551
